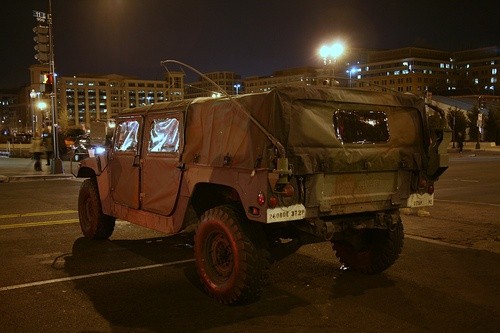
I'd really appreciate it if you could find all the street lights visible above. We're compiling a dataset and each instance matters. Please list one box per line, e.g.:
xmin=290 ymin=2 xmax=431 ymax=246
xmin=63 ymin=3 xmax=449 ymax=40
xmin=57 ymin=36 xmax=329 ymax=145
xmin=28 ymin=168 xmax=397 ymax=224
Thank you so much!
xmin=30 ymin=89 xmax=37 ymax=136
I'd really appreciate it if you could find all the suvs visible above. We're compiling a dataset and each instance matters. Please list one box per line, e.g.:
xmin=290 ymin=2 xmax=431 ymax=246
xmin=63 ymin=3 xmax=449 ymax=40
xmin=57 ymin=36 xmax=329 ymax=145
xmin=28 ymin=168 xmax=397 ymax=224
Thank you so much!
xmin=70 ymin=59 xmax=454 ymax=306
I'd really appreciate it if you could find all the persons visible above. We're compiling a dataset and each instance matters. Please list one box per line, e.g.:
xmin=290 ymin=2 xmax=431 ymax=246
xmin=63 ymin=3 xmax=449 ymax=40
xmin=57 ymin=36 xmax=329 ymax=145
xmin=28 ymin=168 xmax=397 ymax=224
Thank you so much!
xmin=31 ymin=132 xmax=44 ymax=171
xmin=0 ymin=128 xmax=90 ymax=160
xmin=42 ymin=130 xmax=54 ymax=166
xmin=402 ymin=191 xmax=436 ymax=216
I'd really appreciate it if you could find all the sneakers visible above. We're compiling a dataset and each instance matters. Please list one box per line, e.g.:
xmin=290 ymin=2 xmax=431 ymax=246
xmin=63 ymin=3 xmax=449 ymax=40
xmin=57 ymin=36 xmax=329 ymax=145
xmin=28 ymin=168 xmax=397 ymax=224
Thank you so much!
xmin=405 ymin=208 xmax=418 ymax=215
xmin=418 ymin=210 xmax=430 ymax=217
